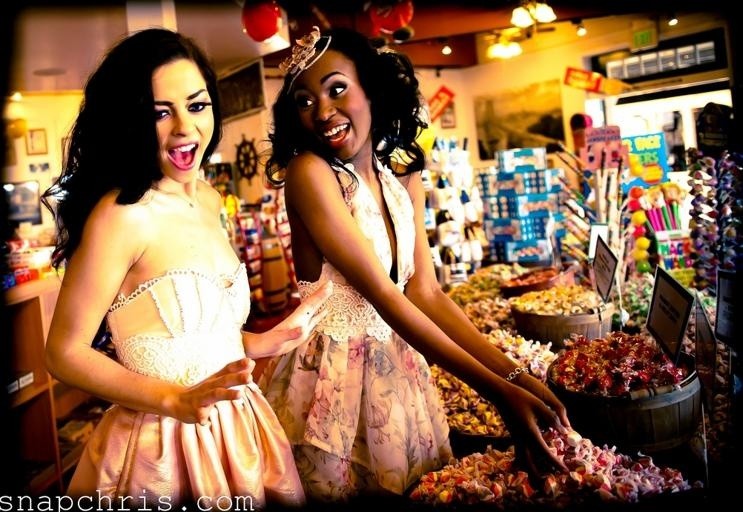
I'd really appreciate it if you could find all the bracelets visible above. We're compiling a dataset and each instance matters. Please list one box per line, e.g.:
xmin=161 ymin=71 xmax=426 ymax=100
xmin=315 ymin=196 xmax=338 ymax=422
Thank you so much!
xmin=505 ymin=366 xmax=530 ymax=382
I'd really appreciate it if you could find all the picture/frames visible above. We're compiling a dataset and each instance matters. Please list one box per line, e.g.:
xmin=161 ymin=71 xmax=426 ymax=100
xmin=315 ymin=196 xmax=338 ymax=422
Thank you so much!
xmin=25 ymin=128 xmax=48 ymax=156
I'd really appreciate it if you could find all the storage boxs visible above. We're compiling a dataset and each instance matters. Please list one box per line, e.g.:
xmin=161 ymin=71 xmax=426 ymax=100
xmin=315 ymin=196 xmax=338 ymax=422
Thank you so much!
xmin=477 ymin=144 xmax=567 ymax=267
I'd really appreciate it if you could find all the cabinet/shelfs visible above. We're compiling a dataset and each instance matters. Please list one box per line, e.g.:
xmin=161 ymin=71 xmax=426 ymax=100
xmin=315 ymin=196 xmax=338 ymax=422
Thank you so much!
xmin=4 ymin=268 xmax=121 ymax=512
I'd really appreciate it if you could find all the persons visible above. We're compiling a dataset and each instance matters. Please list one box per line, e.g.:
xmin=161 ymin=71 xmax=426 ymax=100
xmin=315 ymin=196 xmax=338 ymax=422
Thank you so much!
xmin=41 ymin=26 xmax=335 ymax=510
xmin=255 ymin=24 xmax=573 ymax=503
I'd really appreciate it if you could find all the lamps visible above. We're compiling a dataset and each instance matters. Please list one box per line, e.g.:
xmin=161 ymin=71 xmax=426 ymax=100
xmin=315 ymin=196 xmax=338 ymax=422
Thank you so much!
xmin=481 ymin=28 xmax=528 ymax=60
xmin=509 ymin=0 xmax=559 ymax=29
xmin=571 ymin=19 xmax=587 ymax=38
